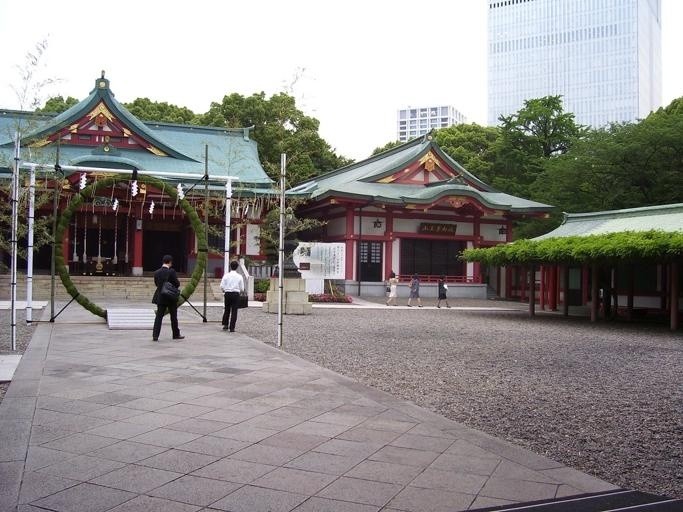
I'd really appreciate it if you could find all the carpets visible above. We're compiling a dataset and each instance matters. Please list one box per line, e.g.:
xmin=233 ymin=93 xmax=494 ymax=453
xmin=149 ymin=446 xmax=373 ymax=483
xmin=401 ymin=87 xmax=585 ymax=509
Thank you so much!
xmin=455 ymin=491 xmax=682 ymax=511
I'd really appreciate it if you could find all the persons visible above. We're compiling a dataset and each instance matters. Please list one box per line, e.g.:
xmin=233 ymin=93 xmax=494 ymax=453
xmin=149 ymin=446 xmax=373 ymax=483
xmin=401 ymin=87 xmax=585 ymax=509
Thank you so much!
xmin=407 ymin=273 xmax=423 ymax=308
xmin=219 ymin=260 xmax=243 ymax=334
xmin=385 ymin=272 xmax=399 ymax=307
xmin=435 ymin=275 xmax=451 ymax=309
xmin=152 ymin=254 xmax=185 ymax=341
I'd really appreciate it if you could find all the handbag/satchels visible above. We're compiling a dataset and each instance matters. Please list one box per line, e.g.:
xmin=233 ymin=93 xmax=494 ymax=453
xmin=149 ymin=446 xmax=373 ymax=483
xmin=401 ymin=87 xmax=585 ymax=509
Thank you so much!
xmin=443 ymin=281 xmax=448 ymax=290
xmin=386 ymin=286 xmax=391 ymax=293
xmin=408 ymin=279 xmax=413 ymax=288
xmin=161 ymin=268 xmax=181 ymax=302
xmin=235 ymin=290 xmax=249 ymax=309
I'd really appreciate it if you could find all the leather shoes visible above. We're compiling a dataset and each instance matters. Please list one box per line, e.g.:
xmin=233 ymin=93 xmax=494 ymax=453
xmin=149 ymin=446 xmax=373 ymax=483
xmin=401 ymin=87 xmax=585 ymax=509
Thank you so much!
xmin=223 ymin=326 xmax=228 ymax=330
xmin=173 ymin=336 xmax=185 ymax=340
xmin=153 ymin=336 xmax=158 ymax=341
xmin=386 ymin=302 xmax=451 ymax=309
xmin=230 ymin=330 xmax=235 ymax=332
